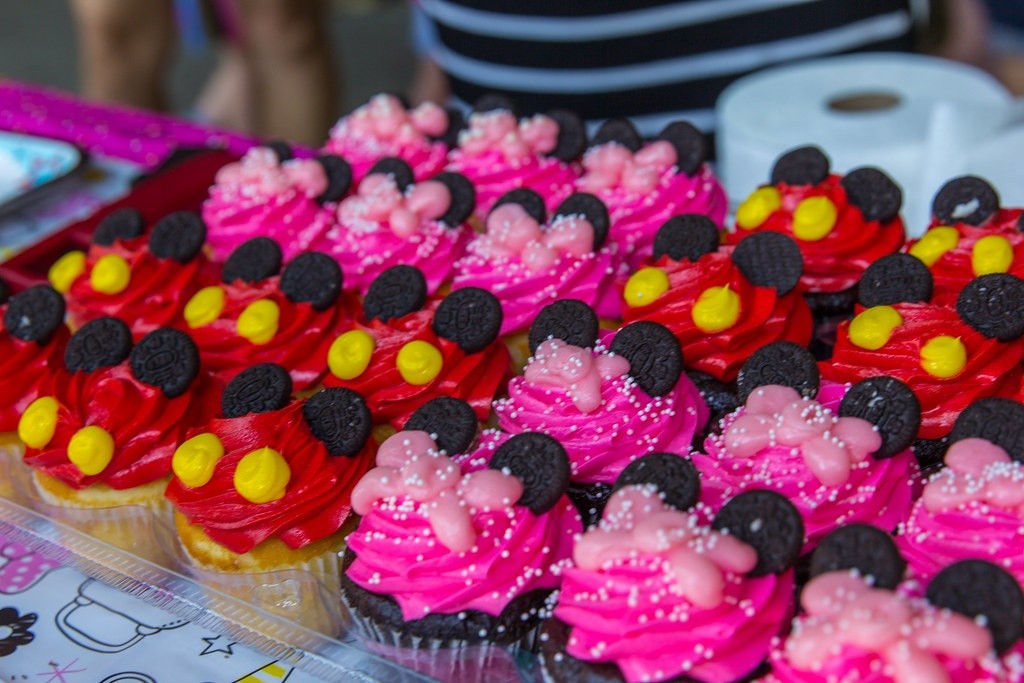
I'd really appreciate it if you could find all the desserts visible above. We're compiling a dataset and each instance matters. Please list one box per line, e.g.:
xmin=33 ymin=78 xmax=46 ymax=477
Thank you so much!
xmin=0 ymin=92 xmax=1024 ymax=683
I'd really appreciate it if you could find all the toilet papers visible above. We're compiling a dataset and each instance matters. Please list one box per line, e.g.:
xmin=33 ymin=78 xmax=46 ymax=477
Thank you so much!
xmin=714 ymin=52 xmax=1024 ymax=239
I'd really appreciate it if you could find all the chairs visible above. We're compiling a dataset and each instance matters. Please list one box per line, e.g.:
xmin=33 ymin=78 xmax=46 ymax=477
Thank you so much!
xmin=714 ymin=49 xmax=1016 ymax=205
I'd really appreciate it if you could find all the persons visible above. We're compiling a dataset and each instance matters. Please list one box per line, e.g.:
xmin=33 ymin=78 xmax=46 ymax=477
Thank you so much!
xmin=417 ymin=1 xmax=992 ymax=180
xmin=70 ymin=0 xmax=338 ymax=150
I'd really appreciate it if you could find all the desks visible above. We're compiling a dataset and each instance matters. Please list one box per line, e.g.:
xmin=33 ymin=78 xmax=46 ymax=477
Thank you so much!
xmin=0 ymin=76 xmax=311 ymax=683
